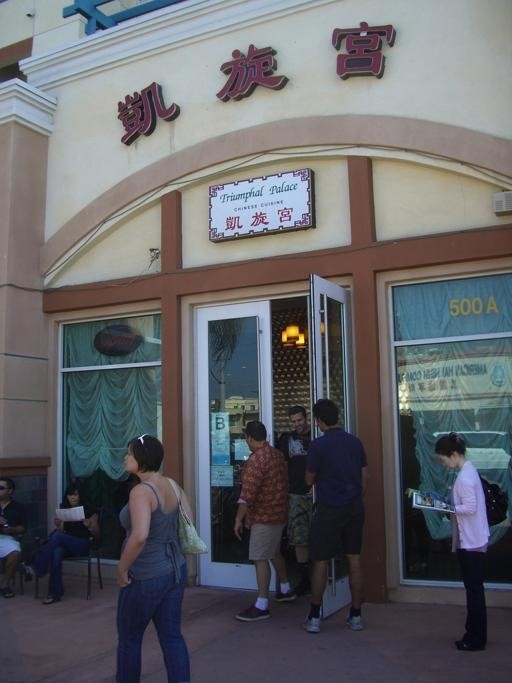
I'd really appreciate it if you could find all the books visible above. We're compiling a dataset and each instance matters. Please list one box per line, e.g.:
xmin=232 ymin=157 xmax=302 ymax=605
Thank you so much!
xmin=404 ymin=487 xmax=456 ymax=514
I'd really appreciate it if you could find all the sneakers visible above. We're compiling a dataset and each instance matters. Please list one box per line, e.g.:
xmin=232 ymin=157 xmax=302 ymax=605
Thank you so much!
xmin=346 ymin=614 xmax=363 ymax=631
xmin=454 ymin=640 xmax=486 ymax=651
xmin=236 ymin=605 xmax=271 ymax=622
xmin=273 ymin=589 xmax=296 ymax=602
xmin=303 ymin=615 xmax=322 ymax=633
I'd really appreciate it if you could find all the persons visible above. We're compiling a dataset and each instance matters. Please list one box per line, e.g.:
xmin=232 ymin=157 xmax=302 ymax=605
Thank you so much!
xmin=433 ymin=431 xmax=491 ymax=651
xmin=18 ymin=480 xmax=101 ymax=604
xmin=0 ymin=476 xmax=29 ymax=598
xmin=114 ymin=433 xmax=194 ymax=682
xmin=234 ymin=398 xmax=369 ymax=633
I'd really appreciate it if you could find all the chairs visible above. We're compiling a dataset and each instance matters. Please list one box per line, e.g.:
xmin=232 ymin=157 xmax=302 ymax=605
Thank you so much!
xmin=11 ymin=533 xmax=24 ymax=595
xmin=34 ymin=507 xmax=103 ymax=600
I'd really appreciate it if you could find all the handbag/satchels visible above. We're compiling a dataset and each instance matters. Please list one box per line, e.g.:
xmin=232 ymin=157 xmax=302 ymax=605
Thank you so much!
xmin=178 ymin=513 xmax=208 ymax=555
xmin=481 ymin=480 xmax=507 ymax=526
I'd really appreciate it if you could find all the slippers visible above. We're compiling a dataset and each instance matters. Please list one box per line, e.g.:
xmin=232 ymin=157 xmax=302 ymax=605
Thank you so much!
xmin=43 ymin=596 xmax=60 ymax=604
xmin=1 ymin=587 xmax=14 ymax=597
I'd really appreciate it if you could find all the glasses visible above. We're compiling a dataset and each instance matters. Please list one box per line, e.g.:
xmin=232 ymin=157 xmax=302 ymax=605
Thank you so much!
xmin=137 ymin=434 xmax=146 ymax=445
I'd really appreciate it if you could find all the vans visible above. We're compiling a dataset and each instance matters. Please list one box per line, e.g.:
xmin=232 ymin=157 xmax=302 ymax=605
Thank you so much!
xmin=433 ymin=431 xmax=510 ymax=482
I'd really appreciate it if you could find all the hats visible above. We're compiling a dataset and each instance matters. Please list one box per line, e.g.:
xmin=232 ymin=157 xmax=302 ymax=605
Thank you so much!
xmin=242 ymin=420 xmax=266 ymax=441
